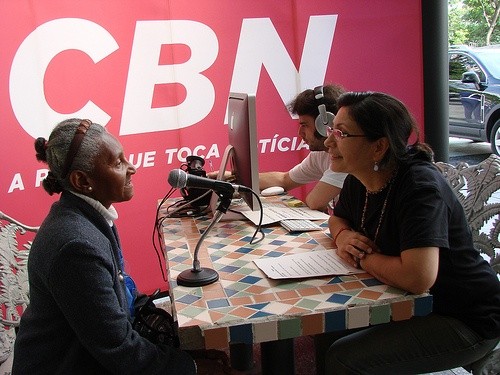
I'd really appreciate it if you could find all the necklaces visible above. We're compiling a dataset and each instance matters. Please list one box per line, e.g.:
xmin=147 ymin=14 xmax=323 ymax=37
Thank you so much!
xmin=362 ymin=167 xmax=397 ymax=247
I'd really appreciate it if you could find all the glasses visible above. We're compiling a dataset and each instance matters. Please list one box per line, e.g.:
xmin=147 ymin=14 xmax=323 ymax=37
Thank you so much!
xmin=326 ymin=125 xmax=367 ymax=140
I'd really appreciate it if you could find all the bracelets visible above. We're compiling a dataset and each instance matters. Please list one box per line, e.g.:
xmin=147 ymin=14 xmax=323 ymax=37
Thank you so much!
xmin=356 ymin=257 xmax=361 ymax=267
xmin=335 ymin=227 xmax=352 ymax=243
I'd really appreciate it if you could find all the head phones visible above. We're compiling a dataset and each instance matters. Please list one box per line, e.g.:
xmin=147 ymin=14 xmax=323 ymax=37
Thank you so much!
xmin=313 ymin=86 xmax=335 ymax=137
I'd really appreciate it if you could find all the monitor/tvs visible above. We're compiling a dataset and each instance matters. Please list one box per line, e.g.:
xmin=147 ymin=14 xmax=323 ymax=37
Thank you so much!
xmin=210 ymin=92 xmax=260 ymax=222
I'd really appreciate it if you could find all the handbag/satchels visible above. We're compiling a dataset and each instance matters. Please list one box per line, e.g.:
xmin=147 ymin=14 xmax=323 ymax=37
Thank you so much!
xmin=133 ymin=289 xmax=176 ymax=352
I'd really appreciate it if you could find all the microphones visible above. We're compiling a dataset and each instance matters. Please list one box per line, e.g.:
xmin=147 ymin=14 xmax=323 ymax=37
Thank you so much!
xmin=167 ymin=169 xmax=251 ymax=193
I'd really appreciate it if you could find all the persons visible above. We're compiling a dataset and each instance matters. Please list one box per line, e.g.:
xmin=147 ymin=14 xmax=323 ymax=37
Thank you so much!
xmin=11 ymin=118 xmax=233 ymax=375
xmin=323 ymin=90 xmax=500 ymax=375
xmin=207 ymin=84 xmax=350 ymax=210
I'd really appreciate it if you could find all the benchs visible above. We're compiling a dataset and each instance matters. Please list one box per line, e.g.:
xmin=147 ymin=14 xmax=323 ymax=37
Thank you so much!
xmin=433 ymin=154 xmax=500 ymax=375
xmin=0 ymin=211 xmax=41 ymax=375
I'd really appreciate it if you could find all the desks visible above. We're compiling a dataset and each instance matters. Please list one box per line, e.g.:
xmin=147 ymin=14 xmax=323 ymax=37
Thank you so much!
xmin=156 ymin=193 xmax=434 ymax=375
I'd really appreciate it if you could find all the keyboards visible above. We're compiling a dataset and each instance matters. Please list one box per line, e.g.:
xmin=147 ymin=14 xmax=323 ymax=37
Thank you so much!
xmin=261 ymin=203 xmax=323 ymax=233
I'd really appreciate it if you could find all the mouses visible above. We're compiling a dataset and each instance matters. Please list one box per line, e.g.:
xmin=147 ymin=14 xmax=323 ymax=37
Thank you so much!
xmin=261 ymin=186 xmax=284 ymax=196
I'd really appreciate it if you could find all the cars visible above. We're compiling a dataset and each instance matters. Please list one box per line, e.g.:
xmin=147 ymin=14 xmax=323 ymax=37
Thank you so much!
xmin=450 ymin=45 xmax=500 ymax=157
